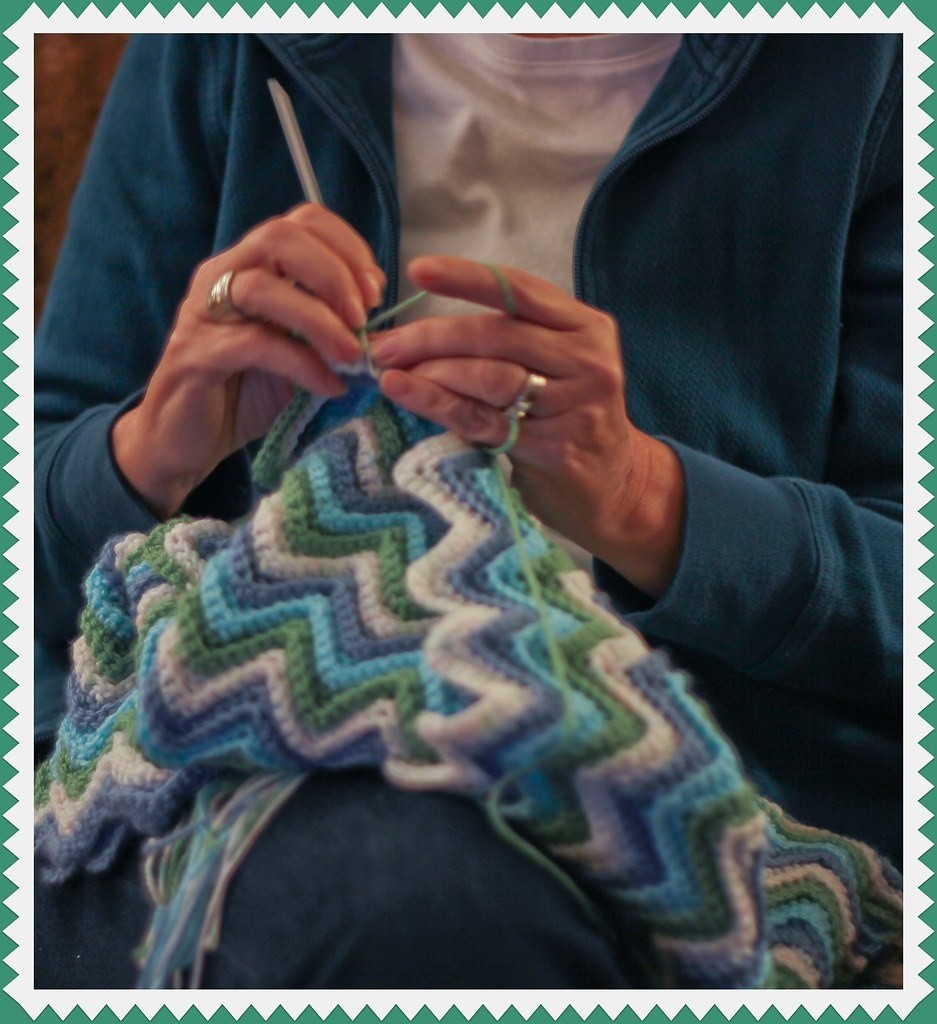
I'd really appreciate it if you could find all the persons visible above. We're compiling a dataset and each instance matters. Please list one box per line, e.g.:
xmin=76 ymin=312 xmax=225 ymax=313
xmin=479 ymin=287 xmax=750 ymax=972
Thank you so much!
xmin=33 ymin=32 xmax=904 ymax=990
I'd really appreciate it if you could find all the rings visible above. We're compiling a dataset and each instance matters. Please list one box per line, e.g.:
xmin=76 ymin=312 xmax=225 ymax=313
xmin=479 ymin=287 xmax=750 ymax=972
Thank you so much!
xmin=206 ymin=263 xmax=257 ymax=325
xmin=501 ymin=369 xmax=548 ymax=422
